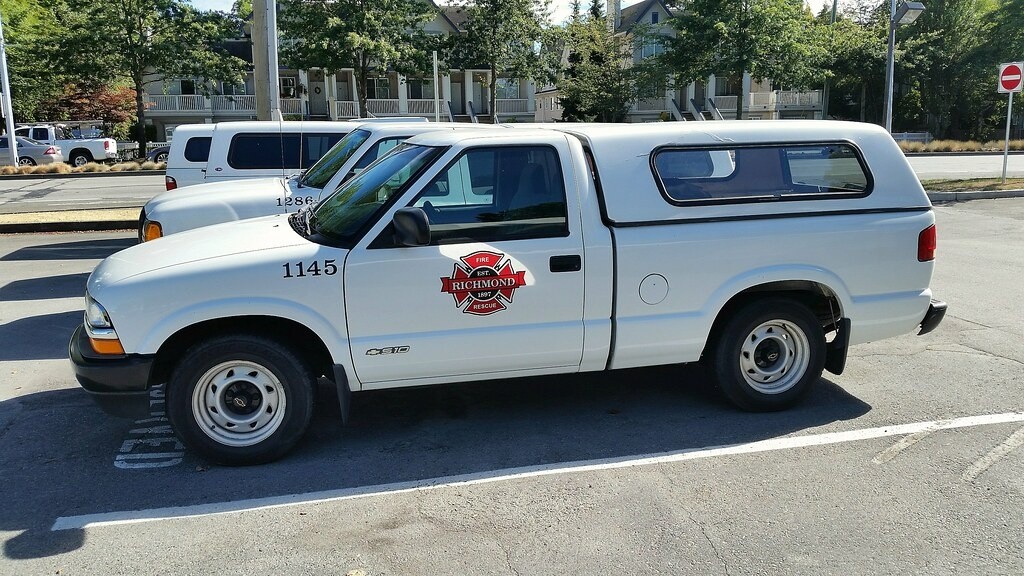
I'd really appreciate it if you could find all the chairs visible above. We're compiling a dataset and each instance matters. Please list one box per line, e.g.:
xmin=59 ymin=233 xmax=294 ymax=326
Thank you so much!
xmin=509 ymin=163 xmax=565 ymax=209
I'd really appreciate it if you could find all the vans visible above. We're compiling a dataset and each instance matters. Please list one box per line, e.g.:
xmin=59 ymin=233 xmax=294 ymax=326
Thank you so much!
xmin=166 ymin=123 xmax=217 ymax=191
xmin=202 ymin=121 xmax=378 ymax=183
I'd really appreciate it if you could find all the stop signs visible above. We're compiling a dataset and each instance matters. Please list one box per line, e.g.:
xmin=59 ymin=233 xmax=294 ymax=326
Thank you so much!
xmin=998 ymin=62 xmax=1024 ymax=93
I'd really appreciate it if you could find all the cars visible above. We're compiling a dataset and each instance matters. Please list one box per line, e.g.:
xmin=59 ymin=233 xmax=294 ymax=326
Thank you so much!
xmin=0 ymin=136 xmax=63 ymax=167
xmin=147 ymin=145 xmax=170 ymax=166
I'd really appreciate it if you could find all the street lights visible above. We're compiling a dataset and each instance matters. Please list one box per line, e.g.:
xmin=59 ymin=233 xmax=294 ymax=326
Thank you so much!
xmin=882 ymin=2 xmax=926 ymax=136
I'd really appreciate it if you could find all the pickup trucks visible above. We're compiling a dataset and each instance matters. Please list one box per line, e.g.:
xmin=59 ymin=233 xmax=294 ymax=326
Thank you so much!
xmin=138 ymin=120 xmax=619 ymax=245
xmin=15 ymin=125 xmax=118 ymax=167
xmin=69 ymin=120 xmax=948 ymax=467
